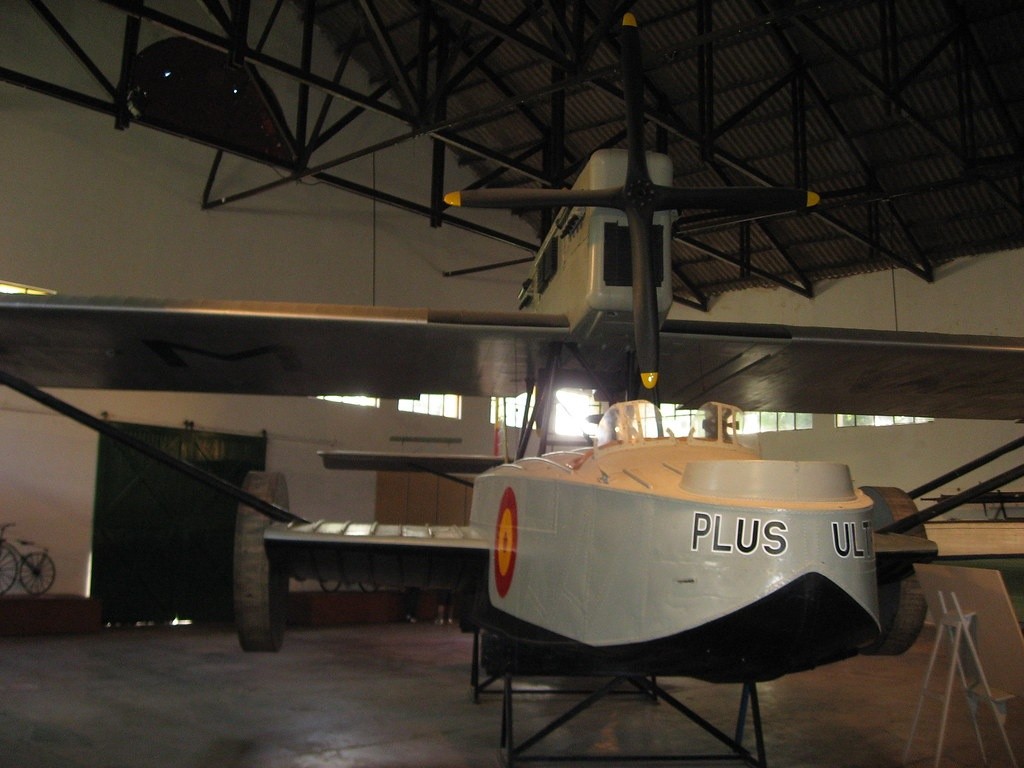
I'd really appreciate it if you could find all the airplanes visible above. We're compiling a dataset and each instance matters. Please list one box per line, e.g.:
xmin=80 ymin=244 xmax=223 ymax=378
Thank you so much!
xmin=3 ymin=13 xmax=1021 ymax=768
